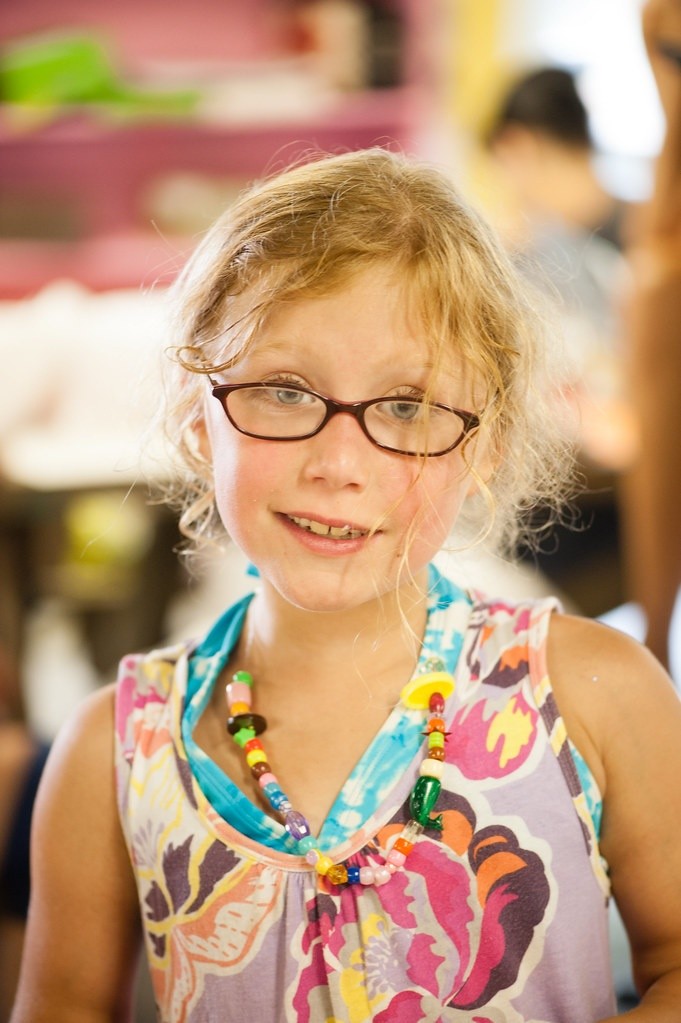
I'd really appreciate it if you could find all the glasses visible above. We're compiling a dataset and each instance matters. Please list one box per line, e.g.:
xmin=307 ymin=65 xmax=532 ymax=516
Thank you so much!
xmin=201 ymin=361 xmax=484 ymax=459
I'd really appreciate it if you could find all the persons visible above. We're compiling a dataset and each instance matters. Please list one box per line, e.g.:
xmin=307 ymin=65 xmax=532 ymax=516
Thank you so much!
xmin=460 ymin=0 xmax=681 ymax=689
xmin=10 ymin=150 xmax=681 ymax=1023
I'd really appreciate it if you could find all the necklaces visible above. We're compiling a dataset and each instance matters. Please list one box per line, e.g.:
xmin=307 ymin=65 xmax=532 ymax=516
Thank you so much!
xmin=223 ymin=652 xmax=455 ymax=886
